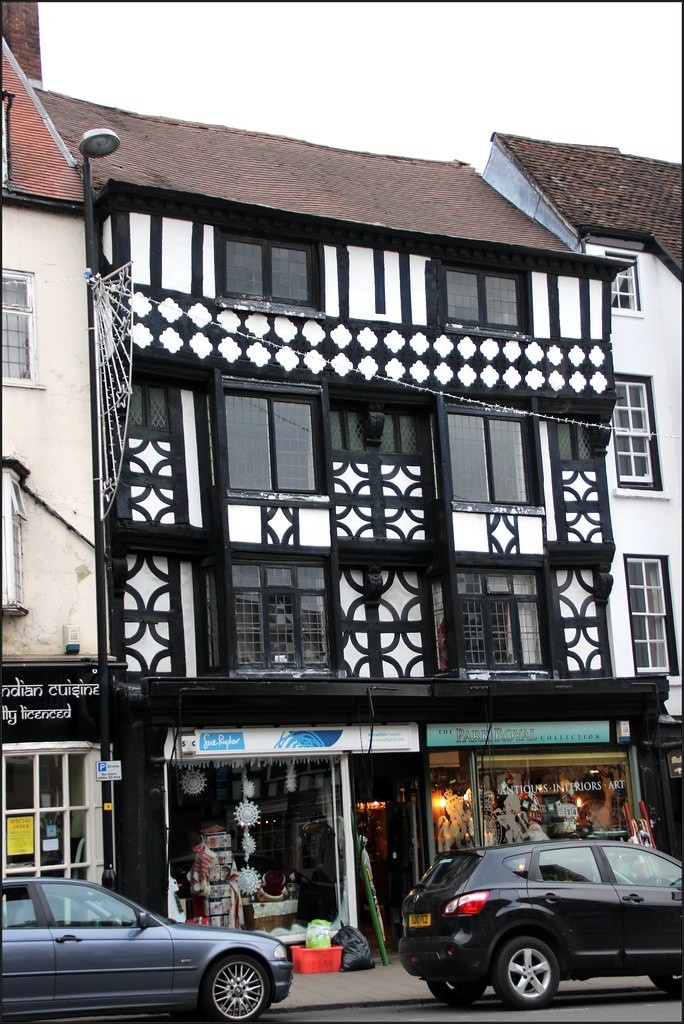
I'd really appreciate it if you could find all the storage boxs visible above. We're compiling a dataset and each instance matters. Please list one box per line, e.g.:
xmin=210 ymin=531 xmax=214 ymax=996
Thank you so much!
xmin=291 ymin=944 xmax=343 ymax=972
xmin=243 ymin=898 xmax=299 ymax=932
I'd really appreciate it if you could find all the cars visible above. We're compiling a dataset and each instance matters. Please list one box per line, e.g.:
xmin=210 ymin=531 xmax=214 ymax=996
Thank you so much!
xmin=1 ymin=875 xmax=292 ymax=1021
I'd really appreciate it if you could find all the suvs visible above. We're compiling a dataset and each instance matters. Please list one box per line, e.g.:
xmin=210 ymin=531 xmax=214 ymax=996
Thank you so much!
xmin=397 ymin=838 xmax=684 ymax=1008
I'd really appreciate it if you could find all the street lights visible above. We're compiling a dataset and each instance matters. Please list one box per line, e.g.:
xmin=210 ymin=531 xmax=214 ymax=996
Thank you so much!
xmin=80 ymin=125 xmax=125 ymax=889
